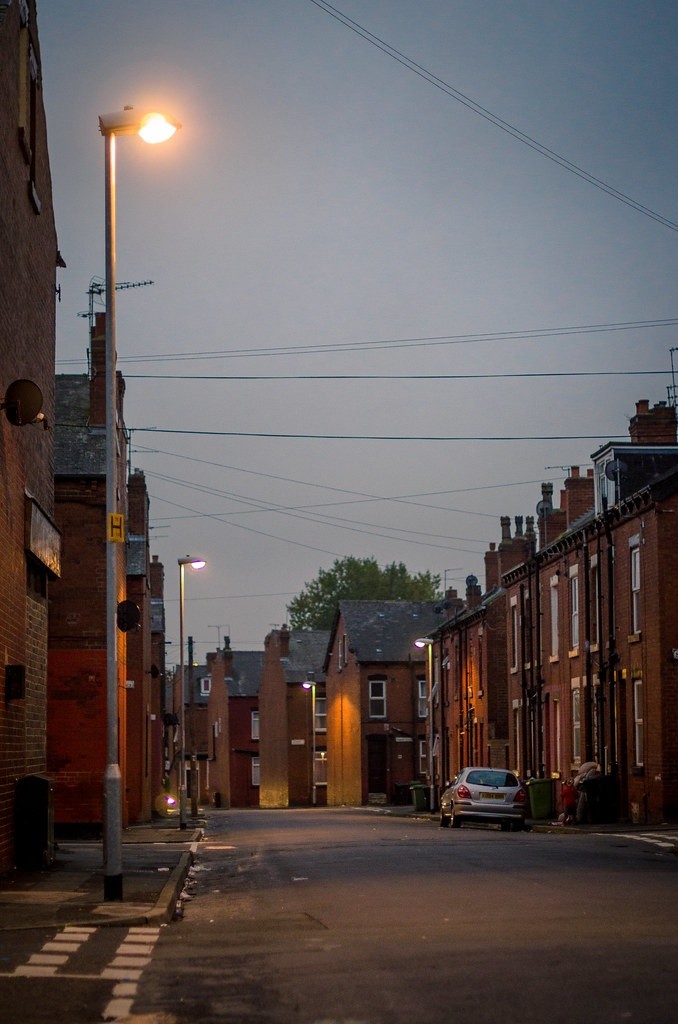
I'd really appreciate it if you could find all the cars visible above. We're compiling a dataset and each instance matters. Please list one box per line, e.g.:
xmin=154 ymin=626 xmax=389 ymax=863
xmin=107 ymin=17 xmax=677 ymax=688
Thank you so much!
xmin=440 ymin=766 xmax=527 ymax=832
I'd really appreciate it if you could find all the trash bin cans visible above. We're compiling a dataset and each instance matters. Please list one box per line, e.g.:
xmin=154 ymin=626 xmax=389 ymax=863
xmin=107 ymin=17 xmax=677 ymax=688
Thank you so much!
xmin=519 ymin=777 xmax=554 ymax=820
xmin=210 ymin=792 xmax=221 ymax=808
xmin=394 ymin=780 xmax=439 ymax=812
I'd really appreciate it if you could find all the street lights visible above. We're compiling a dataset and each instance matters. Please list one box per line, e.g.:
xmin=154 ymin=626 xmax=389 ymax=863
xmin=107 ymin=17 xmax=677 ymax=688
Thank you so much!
xmin=413 ymin=637 xmax=437 ymax=817
xmin=97 ymin=101 xmax=180 ymax=898
xmin=302 ymin=680 xmax=319 ymax=806
xmin=176 ymin=550 xmax=205 ymax=832
